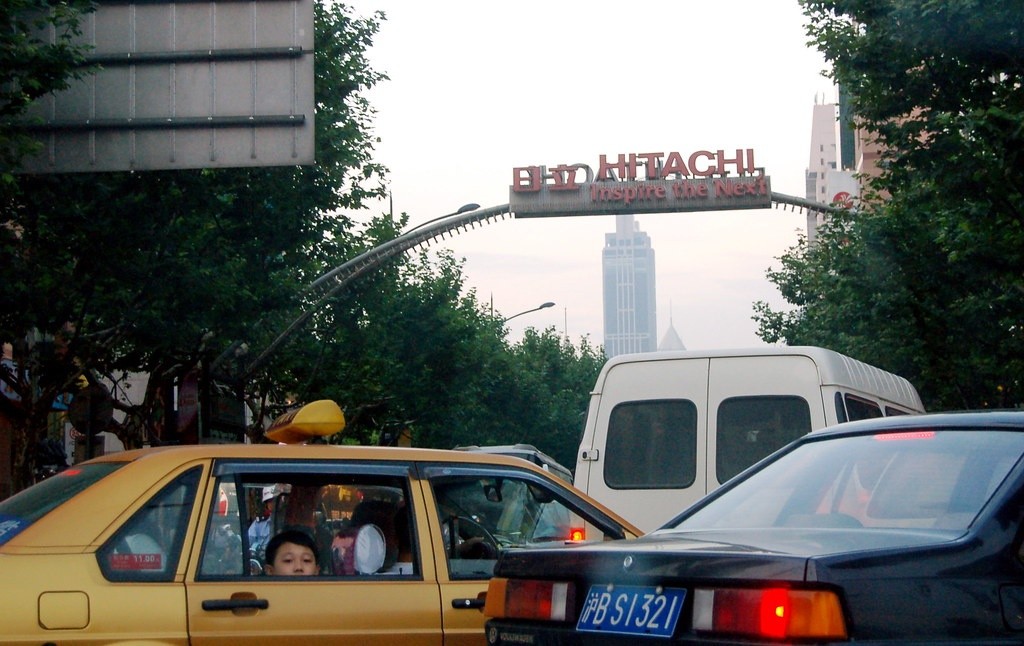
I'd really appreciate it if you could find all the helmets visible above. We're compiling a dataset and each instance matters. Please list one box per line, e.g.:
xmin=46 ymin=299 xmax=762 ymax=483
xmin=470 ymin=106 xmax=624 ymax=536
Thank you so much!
xmin=262 ymin=484 xmax=285 ymax=504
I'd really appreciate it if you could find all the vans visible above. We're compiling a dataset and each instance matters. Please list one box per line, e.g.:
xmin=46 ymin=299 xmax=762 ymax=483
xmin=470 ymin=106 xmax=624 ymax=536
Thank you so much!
xmin=578 ymin=349 xmax=929 ymax=541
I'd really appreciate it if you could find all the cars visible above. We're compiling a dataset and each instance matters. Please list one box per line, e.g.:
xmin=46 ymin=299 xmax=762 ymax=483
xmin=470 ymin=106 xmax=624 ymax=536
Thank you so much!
xmin=1 ymin=400 xmax=653 ymax=646
xmin=482 ymin=410 xmax=1024 ymax=646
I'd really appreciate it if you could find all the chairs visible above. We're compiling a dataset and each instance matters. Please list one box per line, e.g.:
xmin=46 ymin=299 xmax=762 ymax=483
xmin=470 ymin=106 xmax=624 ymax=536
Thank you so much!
xmin=331 ymin=523 xmax=386 ymax=576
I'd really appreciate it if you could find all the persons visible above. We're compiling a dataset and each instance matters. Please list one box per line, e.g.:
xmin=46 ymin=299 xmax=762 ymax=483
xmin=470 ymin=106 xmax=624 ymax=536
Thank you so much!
xmin=349 ymin=498 xmax=484 ymax=574
xmin=247 ymin=485 xmax=277 ymax=561
xmin=265 ymin=533 xmax=320 ymax=576
